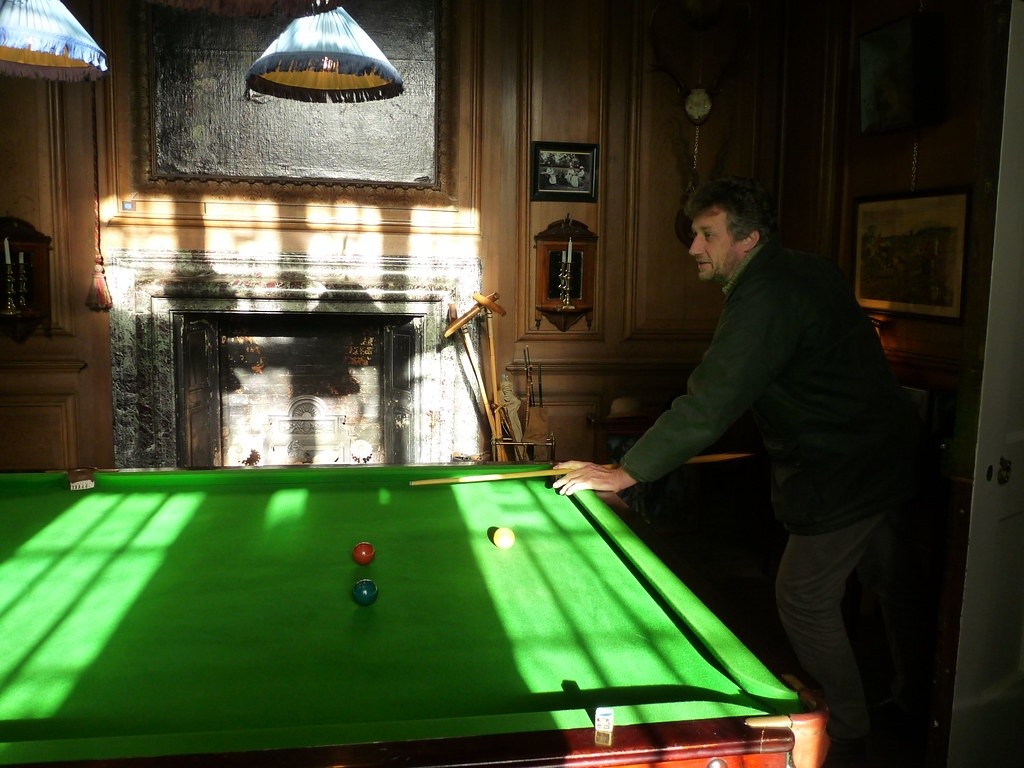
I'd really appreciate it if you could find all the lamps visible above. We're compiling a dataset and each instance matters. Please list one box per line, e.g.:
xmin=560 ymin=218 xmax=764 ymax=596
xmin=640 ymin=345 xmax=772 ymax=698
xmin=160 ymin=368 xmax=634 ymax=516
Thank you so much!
xmin=0 ymin=0 xmax=109 ymax=83
xmin=244 ymin=0 xmax=406 ymax=104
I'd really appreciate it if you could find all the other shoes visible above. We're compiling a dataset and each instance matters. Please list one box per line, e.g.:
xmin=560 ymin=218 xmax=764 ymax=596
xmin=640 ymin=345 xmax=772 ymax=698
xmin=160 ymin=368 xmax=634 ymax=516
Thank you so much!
xmin=865 ymin=697 xmax=929 ymax=750
xmin=830 ymin=737 xmax=867 ymax=763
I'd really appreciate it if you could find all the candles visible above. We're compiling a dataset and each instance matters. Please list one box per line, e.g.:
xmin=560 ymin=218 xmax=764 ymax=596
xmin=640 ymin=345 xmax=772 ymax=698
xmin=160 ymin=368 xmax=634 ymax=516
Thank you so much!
xmin=562 ymin=251 xmax=566 ymax=263
xmin=567 ymin=237 xmax=572 ymax=263
xmin=4 ymin=238 xmax=11 ymax=264
xmin=19 ymin=252 xmax=24 ymax=263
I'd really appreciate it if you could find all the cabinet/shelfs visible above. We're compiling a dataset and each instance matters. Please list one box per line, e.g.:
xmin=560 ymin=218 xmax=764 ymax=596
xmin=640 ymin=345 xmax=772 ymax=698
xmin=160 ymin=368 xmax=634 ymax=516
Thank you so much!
xmin=585 ymin=413 xmax=708 ymax=535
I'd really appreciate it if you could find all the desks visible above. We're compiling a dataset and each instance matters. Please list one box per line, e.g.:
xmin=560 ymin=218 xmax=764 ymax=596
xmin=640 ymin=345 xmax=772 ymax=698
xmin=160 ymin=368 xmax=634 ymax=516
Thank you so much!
xmin=0 ymin=461 xmax=832 ymax=768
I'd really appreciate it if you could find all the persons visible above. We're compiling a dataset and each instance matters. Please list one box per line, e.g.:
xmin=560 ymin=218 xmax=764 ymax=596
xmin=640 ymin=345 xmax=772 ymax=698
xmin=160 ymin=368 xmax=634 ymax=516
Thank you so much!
xmin=551 ymin=174 xmax=934 ymax=742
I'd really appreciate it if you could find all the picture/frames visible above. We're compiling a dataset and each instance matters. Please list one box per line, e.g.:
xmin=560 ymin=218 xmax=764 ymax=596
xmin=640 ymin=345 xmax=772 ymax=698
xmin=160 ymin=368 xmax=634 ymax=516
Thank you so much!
xmin=133 ymin=0 xmax=451 ymax=202
xmin=848 ymin=182 xmax=975 ymax=327
xmin=529 ymin=142 xmax=598 ymax=203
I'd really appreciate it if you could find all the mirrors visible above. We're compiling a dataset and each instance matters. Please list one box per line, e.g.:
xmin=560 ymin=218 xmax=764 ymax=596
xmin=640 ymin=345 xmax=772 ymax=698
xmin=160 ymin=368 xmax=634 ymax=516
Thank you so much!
xmin=0 ymin=249 xmax=34 ymax=308
xmin=547 ymin=249 xmax=582 ymax=301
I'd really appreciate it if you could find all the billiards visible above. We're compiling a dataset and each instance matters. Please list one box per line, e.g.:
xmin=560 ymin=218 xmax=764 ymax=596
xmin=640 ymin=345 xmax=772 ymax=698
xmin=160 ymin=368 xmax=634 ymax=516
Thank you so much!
xmin=353 ymin=579 xmax=378 ymax=604
xmin=493 ymin=527 xmax=515 ymax=549
xmin=352 ymin=541 xmax=376 ymax=565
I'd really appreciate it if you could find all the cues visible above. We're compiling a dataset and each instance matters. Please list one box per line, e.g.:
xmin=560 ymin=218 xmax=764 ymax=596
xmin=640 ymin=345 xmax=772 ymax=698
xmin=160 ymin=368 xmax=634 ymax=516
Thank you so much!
xmin=409 ymin=453 xmax=751 ymax=488
